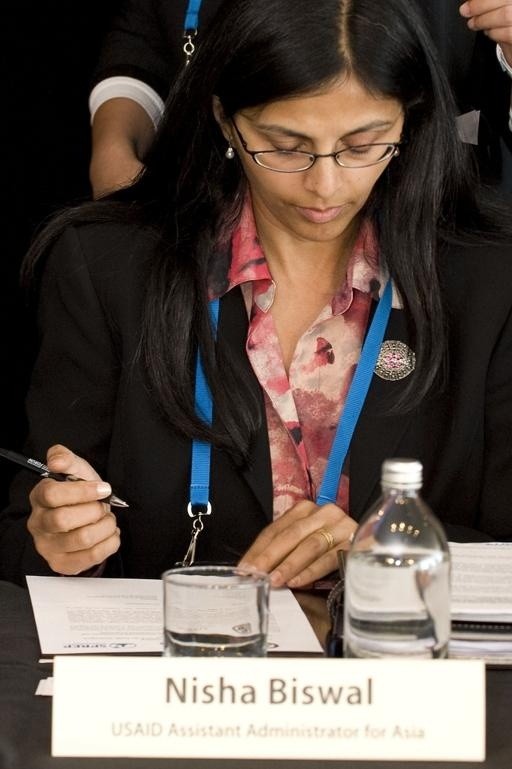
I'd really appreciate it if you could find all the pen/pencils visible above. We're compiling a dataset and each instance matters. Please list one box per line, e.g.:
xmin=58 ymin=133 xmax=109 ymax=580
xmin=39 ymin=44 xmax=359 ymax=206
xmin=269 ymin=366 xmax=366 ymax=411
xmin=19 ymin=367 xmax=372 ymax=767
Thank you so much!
xmin=0 ymin=448 xmax=130 ymax=508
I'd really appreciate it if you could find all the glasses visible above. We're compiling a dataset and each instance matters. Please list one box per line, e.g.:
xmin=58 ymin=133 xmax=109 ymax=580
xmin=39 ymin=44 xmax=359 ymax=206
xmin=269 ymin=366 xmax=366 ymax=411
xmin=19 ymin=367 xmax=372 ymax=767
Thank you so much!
xmin=227 ymin=105 xmax=411 ymax=174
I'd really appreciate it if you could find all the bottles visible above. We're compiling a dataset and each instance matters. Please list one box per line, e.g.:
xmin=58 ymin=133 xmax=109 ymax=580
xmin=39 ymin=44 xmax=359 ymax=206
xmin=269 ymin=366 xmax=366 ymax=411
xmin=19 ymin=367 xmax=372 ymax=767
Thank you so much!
xmin=335 ymin=454 xmax=455 ymax=660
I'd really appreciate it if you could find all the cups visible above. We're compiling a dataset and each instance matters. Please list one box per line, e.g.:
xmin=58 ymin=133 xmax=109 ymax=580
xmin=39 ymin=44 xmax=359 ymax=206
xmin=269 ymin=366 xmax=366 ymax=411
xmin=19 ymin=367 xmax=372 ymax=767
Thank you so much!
xmin=159 ymin=564 xmax=272 ymax=658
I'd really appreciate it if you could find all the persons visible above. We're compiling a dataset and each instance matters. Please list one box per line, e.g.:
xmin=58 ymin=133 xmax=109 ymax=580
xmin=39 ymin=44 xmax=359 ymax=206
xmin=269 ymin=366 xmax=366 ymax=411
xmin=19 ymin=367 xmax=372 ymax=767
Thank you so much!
xmin=79 ymin=0 xmax=511 ymax=245
xmin=4 ymin=6 xmax=511 ymax=593
